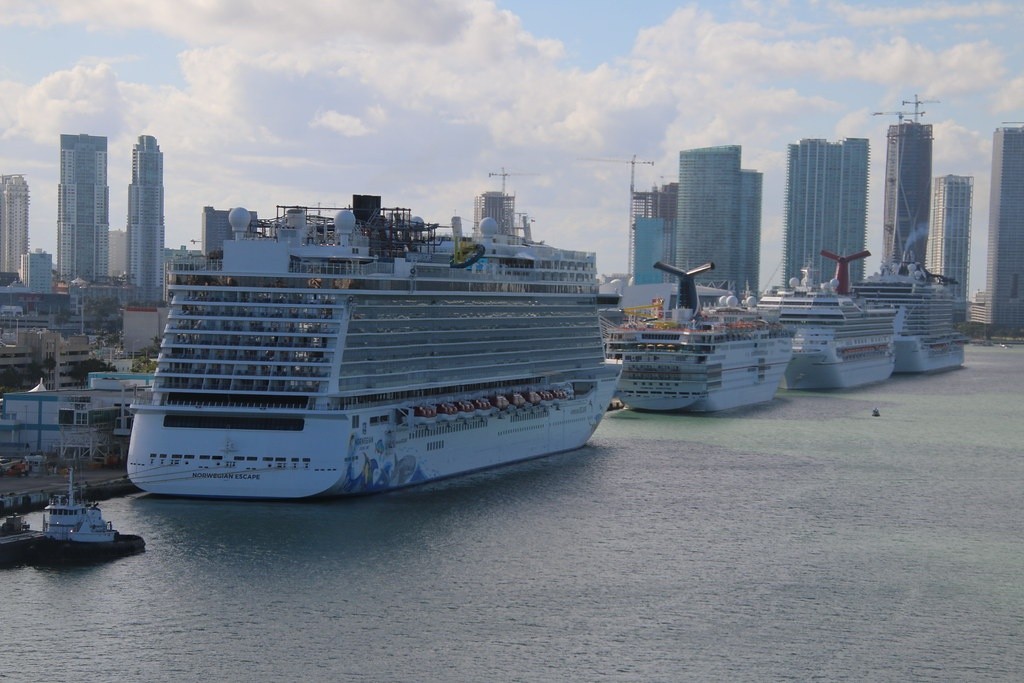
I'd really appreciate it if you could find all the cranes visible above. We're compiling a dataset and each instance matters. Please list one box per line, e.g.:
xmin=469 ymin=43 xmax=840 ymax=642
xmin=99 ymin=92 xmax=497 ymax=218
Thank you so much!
xmin=903 ymin=94 xmax=942 ymax=124
xmin=488 ymin=167 xmax=541 ymax=194
xmin=578 ymin=152 xmax=655 ymax=272
xmin=874 ymin=109 xmax=926 ymax=124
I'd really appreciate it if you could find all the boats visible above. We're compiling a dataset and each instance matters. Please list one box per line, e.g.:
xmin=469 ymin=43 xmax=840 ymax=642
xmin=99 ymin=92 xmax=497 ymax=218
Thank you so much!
xmin=44 ymin=465 xmax=148 ymax=554
xmin=415 ymin=389 xmax=568 ymax=418
xmin=0 ymin=514 xmax=44 ymax=554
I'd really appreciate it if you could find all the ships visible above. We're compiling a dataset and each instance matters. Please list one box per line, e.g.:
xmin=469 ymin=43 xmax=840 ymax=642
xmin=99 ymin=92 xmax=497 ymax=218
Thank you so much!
xmin=604 ymin=260 xmax=792 ymax=413
xmin=850 ymin=249 xmax=971 ymax=374
xmin=756 ymin=250 xmax=898 ymax=390
xmin=125 ymin=194 xmax=627 ymax=500
